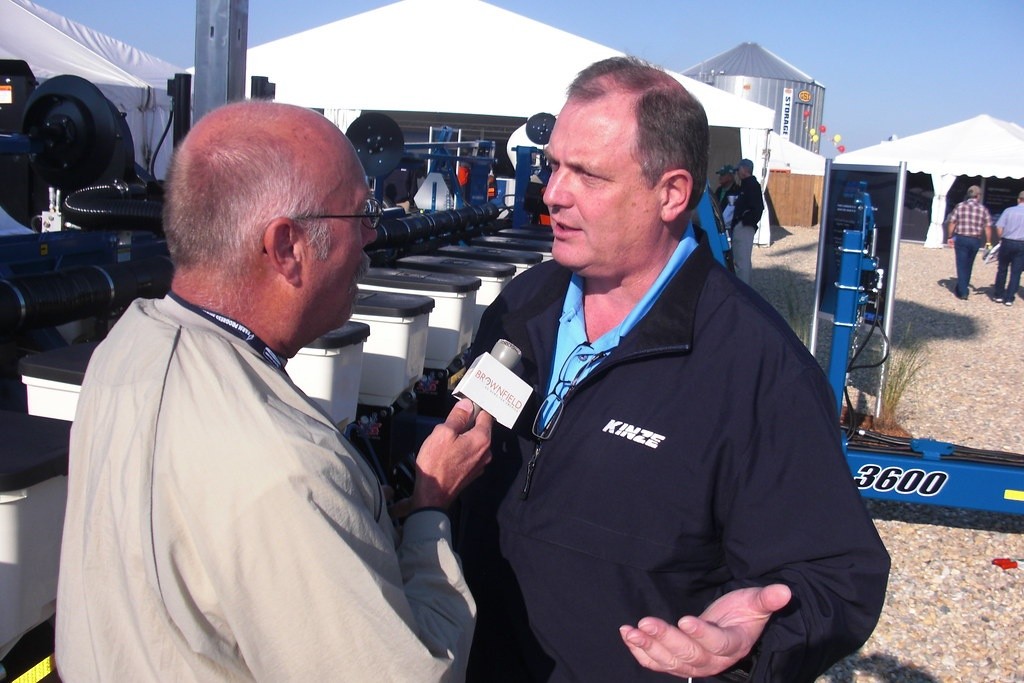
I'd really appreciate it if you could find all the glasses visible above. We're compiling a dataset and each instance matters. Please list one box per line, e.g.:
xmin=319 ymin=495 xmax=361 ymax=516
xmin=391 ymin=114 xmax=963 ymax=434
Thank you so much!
xmin=262 ymin=197 xmax=383 ymax=255
xmin=532 ymin=340 xmax=597 ymax=439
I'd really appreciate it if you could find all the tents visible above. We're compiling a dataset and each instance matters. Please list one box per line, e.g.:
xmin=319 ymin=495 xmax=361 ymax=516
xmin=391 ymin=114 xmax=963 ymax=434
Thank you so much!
xmin=176 ymin=0 xmax=774 ymax=247
xmin=766 ymin=130 xmax=828 ymax=228
xmin=0 ymin=1 xmax=195 ymax=184
xmin=833 ymin=115 xmax=1024 ymax=250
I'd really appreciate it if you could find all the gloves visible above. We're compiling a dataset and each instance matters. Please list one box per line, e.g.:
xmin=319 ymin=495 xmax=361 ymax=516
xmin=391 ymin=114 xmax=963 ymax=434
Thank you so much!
xmin=985 ymin=242 xmax=992 ymax=250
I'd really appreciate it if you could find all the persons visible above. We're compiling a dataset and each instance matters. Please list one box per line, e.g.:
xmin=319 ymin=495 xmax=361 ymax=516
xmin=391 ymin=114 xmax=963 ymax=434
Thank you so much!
xmin=992 ymin=191 xmax=1024 ymax=306
xmin=713 ymin=165 xmax=741 ymax=269
xmin=730 ymin=158 xmax=764 ymax=286
xmin=455 ymin=57 xmax=890 ymax=683
xmin=56 ymin=97 xmax=494 ymax=682
xmin=947 ymin=186 xmax=992 ymax=301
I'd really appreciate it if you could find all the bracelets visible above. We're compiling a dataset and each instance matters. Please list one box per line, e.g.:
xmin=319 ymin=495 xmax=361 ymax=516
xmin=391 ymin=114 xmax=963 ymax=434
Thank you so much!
xmin=947 ymin=236 xmax=953 ymax=239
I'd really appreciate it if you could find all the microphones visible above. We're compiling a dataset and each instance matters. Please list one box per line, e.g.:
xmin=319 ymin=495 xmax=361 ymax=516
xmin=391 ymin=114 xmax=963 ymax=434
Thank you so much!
xmin=451 ymin=338 xmax=534 ymax=430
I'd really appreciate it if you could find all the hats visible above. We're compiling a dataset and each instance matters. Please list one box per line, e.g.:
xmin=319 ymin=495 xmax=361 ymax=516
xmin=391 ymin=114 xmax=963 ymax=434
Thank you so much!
xmin=967 ymin=186 xmax=980 ymax=196
xmin=716 ymin=164 xmax=736 ymax=174
xmin=1019 ymin=191 xmax=1024 ymax=198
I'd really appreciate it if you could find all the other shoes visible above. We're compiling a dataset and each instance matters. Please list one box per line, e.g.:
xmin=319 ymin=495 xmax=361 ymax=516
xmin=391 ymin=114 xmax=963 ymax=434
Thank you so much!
xmin=1006 ymin=301 xmax=1013 ymax=305
xmin=960 ymin=295 xmax=968 ymax=300
xmin=993 ymin=297 xmax=1003 ymax=302
